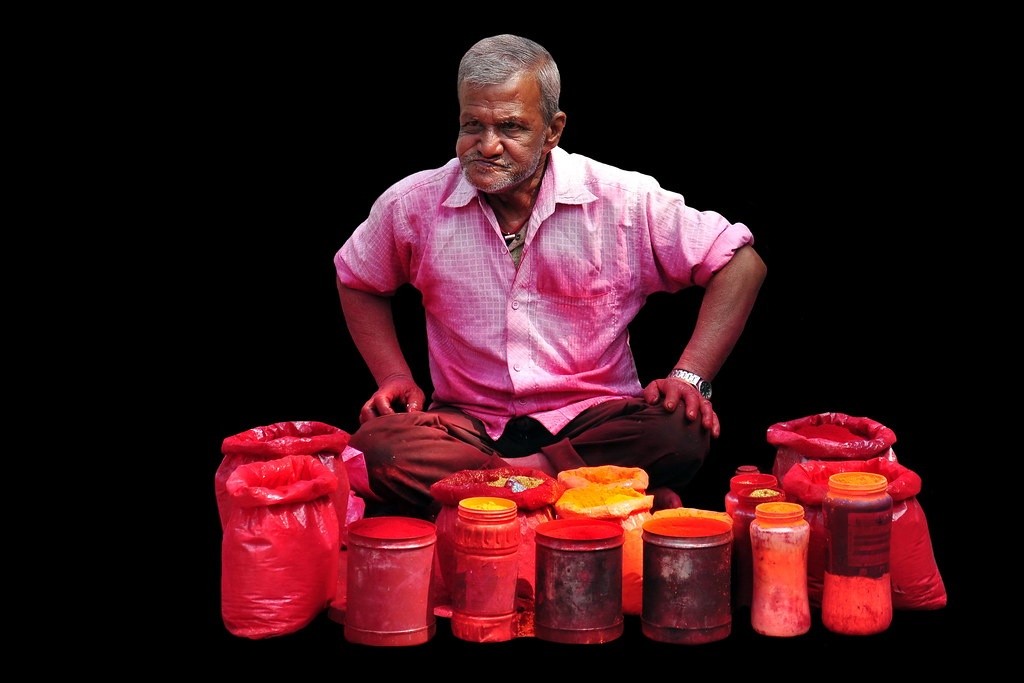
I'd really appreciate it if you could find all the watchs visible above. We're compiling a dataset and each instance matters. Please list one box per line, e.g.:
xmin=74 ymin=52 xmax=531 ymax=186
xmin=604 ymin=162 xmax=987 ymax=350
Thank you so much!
xmin=667 ymin=369 xmax=712 ymax=399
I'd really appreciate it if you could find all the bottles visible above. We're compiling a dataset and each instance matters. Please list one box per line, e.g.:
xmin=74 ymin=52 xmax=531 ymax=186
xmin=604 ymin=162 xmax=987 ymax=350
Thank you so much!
xmin=342 ymin=468 xmax=894 ymax=648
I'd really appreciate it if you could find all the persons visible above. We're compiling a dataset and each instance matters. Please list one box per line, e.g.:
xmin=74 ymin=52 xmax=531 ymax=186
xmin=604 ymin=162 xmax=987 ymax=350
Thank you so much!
xmin=333 ymin=34 xmax=767 ymax=512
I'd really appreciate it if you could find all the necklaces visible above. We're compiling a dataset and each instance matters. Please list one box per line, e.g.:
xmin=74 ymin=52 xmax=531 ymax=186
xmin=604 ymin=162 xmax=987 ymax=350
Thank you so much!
xmin=499 ymin=216 xmax=527 ymax=240
